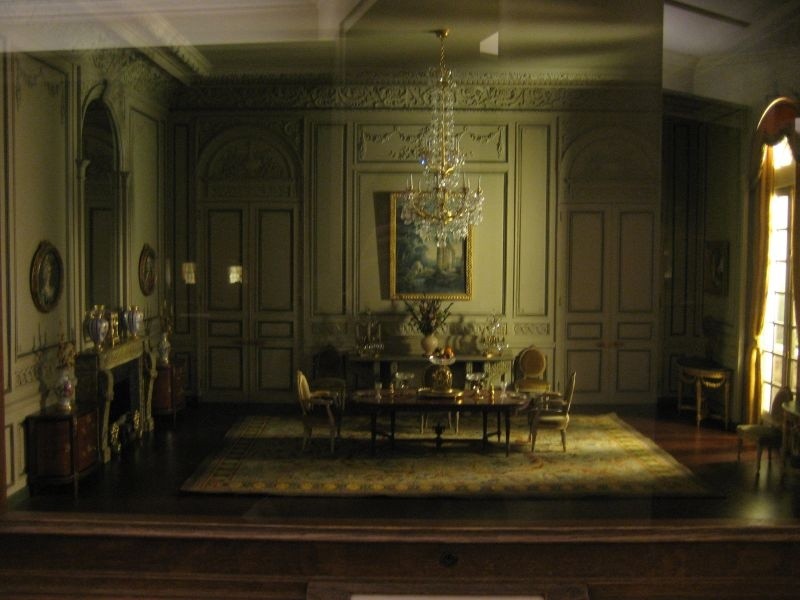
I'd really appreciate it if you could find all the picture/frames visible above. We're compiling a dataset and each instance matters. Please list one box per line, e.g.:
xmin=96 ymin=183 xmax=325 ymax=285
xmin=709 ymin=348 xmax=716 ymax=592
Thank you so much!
xmin=139 ymin=243 xmax=156 ymax=297
xmin=390 ymin=191 xmax=475 ymax=302
xmin=702 ymin=240 xmax=730 ymax=298
xmin=29 ymin=239 xmax=64 ymax=314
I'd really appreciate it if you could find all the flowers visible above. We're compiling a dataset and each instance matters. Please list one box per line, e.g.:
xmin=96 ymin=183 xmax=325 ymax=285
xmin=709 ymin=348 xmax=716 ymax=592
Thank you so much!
xmin=398 ymin=293 xmax=459 ymax=336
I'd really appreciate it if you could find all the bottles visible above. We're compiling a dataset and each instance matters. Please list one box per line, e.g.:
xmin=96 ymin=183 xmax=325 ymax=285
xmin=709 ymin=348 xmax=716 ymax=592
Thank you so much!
xmin=499 ymin=381 xmax=507 ymax=398
xmin=374 ymin=382 xmax=384 ymax=400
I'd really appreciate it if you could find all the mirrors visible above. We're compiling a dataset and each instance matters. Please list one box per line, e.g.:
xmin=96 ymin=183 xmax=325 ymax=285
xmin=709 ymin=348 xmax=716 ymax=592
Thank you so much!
xmin=80 ymin=100 xmax=120 ymax=339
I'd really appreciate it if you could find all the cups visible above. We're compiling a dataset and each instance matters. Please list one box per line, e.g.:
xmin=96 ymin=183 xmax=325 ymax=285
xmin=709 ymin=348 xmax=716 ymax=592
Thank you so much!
xmin=401 ymin=384 xmax=410 ymax=395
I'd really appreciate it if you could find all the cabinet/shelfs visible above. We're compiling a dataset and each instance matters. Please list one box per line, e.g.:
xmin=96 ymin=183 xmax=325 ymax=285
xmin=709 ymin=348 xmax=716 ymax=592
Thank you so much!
xmin=152 ymin=360 xmax=187 ymax=417
xmin=27 ymin=400 xmax=105 ymax=509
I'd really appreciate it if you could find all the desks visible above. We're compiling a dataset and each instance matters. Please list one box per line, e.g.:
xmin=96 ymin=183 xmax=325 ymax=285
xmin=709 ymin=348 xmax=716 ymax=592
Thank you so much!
xmin=348 ymin=353 xmax=514 ymax=418
xmin=351 ymin=387 xmax=527 ymax=461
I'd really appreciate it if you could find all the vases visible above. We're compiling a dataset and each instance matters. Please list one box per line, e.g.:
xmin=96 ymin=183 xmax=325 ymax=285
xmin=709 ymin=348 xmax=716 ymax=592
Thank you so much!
xmin=51 ymin=366 xmax=75 ymax=411
xmin=127 ymin=307 xmax=143 ymax=342
xmin=85 ymin=305 xmax=109 ymax=354
xmin=158 ymin=332 xmax=172 ymax=369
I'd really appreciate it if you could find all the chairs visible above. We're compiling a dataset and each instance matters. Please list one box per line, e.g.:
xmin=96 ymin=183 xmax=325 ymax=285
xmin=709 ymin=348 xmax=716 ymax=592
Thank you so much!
xmin=527 ymin=369 xmax=577 ymax=453
xmin=735 ymin=385 xmax=794 ymax=478
xmin=673 ymin=318 xmax=737 ymax=431
xmin=516 ymin=340 xmax=551 ymax=402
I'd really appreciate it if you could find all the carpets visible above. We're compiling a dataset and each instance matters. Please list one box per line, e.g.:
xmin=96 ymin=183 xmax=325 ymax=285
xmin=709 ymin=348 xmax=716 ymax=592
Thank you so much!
xmin=175 ymin=408 xmax=725 ymax=498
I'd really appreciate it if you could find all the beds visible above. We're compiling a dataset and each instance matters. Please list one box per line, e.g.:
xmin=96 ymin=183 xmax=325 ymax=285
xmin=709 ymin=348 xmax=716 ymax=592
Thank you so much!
xmin=295 ymin=365 xmax=340 ymax=454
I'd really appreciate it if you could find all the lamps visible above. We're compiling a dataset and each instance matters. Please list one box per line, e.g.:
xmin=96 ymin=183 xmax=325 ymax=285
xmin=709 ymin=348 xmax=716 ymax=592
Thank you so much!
xmin=421 ymin=334 xmax=439 ymax=358
xmin=398 ymin=29 xmax=485 ymax=250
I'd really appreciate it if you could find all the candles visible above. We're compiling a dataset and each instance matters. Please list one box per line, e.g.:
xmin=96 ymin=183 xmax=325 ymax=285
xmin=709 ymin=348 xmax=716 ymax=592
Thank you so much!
xmin=356 ymin=324 xmax=359 ymax=337
xmin=368 ymin=324 xmax=370 ymax=341
xmin=378 ymin=324 xmax=381 ymax=337
